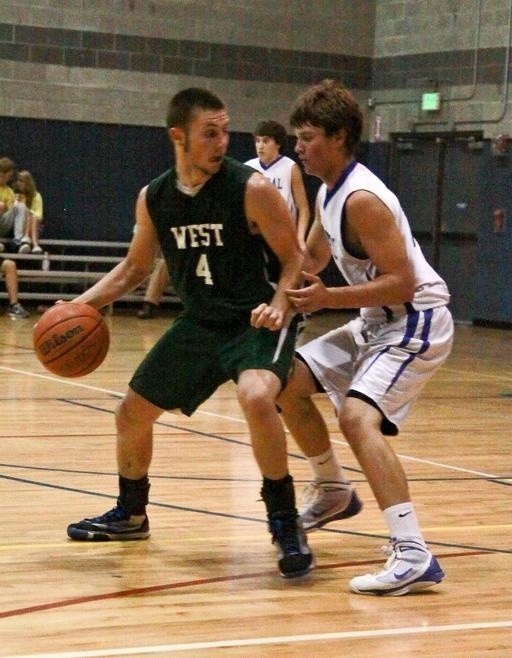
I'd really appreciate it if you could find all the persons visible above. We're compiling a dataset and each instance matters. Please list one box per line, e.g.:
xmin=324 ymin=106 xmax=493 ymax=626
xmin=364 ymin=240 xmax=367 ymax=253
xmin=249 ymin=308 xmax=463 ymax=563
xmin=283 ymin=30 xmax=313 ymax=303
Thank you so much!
xmin=135 ymin=257 xmax=171 ymax=319
xmin=6 ymin=169 xmax=44 ymax=254
xmin=235 ymin=117 xmax=312 ymax=273
xmin=0 ymin=156 xmax=35 ymax=254
xmin=32 ymin=85 xmax=316 ymax=581
xmin=1 ymin=256 xmax=32 ymax=319
xmin=271 ymin=77 xmax=459 ymax=599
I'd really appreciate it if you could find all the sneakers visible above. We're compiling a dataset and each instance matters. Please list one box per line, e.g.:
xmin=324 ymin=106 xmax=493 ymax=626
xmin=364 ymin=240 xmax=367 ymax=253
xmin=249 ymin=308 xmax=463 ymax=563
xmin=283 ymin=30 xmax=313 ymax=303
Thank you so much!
xmin=349 ymin=536 xmax=451 ymax=597
xmin=133 ymin=298 xmax=157 ymax=318
xmin=31 ymin=243 xmax=43 ymax=256
xmin=65 ymin=504 xmax=152 ymax=544
xmin=262 ymin=508 xmax=323 ymax=581
xmin=300 ymin=480 xmax=365 ymax=534
xmin=6 ymin=301 xmax=34 ymax=322
xmin=16 ymin=235 xmax=33 ymax=257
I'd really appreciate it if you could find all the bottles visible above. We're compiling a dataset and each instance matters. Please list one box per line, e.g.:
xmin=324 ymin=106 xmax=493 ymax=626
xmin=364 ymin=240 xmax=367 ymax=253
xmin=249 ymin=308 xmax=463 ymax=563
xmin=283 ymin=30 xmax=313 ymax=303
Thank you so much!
xmin=42 ymin=250 xmax=51 ymax=269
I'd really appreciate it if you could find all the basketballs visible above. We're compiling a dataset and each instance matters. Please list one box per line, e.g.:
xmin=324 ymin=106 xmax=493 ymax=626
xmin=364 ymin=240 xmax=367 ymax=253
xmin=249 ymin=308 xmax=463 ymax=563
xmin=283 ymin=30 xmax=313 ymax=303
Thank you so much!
xmin=33 ymin=302 xmax=109 ymax=377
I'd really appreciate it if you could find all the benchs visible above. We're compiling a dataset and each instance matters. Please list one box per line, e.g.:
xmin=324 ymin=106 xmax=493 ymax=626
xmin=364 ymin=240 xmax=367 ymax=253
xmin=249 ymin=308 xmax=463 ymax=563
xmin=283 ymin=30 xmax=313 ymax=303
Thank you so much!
xmin=1 ymin=234 xmax=182 ymax=314
